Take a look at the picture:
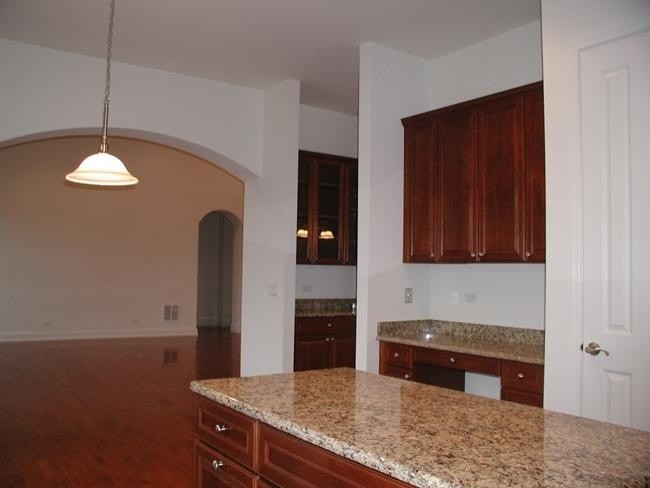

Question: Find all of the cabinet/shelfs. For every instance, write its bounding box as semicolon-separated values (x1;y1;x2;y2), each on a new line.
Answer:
498;353;545;413
296;147;358;268
294;308;357;373
191;395;422;488
521;75;547;267
434;83;526;267
396;107;439;267
376;337;465;393
410;343;502;379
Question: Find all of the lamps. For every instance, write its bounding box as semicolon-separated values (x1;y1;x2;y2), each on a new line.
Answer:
55;1;143;189
295;220;336;242
